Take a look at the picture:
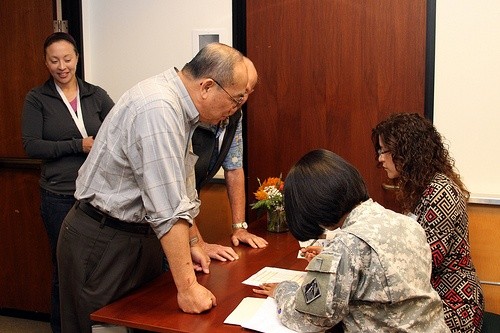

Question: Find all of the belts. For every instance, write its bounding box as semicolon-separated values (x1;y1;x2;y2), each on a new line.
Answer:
42;189;75;199
78;200;155;234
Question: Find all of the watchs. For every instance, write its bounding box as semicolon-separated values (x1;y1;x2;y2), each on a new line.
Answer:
232;222;248;231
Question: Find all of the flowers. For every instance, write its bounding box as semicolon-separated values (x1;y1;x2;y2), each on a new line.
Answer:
250;173;284;212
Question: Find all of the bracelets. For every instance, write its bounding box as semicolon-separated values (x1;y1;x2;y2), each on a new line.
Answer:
189;235;199;246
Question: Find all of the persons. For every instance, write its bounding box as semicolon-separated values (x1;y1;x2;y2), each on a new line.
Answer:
371;110;485;333
21;31;115;333
190;55;270;262
252;149;451;333
56;42;248;333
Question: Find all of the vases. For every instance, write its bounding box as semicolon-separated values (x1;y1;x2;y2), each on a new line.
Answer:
266;210;289;232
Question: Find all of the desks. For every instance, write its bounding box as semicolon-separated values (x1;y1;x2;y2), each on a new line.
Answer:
90;215;326;333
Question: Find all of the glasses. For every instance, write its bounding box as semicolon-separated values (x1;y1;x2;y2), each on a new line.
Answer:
377;149;392;156
200;77;242;109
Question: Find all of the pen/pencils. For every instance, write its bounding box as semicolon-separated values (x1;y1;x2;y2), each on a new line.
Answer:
301;239;317;256
